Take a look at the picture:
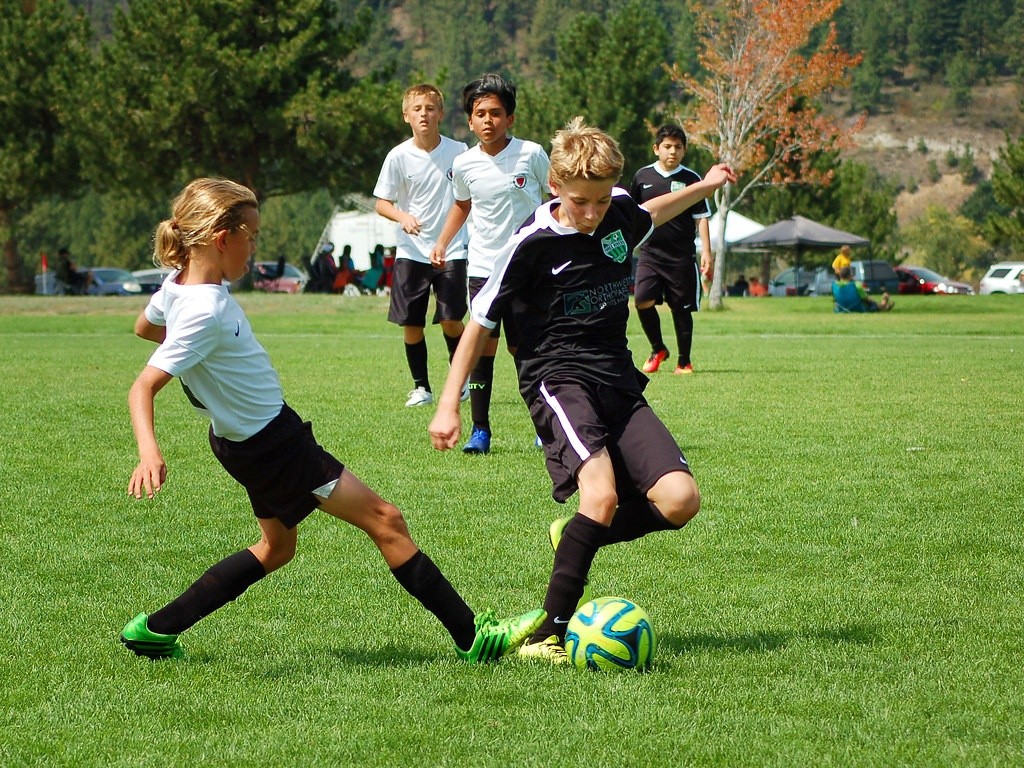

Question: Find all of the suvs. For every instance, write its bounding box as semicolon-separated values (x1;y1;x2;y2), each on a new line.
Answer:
979;260;1024;294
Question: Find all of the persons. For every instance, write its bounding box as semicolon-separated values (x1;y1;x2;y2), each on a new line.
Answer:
119;176;550;663
314;242;385;292
832;268;895;311
832;245;851;276
430;73;554;453
428;115;738;666
371;84;472;408
629;124;703;374
278;254;285;276
726;275;768;297
56;248;94;295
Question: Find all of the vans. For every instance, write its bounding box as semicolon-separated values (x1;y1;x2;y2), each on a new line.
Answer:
811;259;901;294
34;268;142;295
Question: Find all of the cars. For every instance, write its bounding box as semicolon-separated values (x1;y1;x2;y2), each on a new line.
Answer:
253;262;308;295
894;266;976;295
768;258;818;297
130;267;175;285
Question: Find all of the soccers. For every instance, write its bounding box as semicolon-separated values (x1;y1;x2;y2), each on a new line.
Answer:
564;596;657;672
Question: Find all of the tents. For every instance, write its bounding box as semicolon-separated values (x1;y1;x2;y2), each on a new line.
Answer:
694;208;766;283
728;214;874;296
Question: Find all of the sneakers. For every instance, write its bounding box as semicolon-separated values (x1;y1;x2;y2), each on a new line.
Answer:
675;363;693;375
120;613;185;659
641;346;669;373
405;386;432;408
517;633;569;664
461;373;471;402
455;609;548;664
462;427;489;454
550;515;591;613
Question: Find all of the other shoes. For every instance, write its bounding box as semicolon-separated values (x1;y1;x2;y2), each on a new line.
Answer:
885;300;895;311
881;293;889;305
